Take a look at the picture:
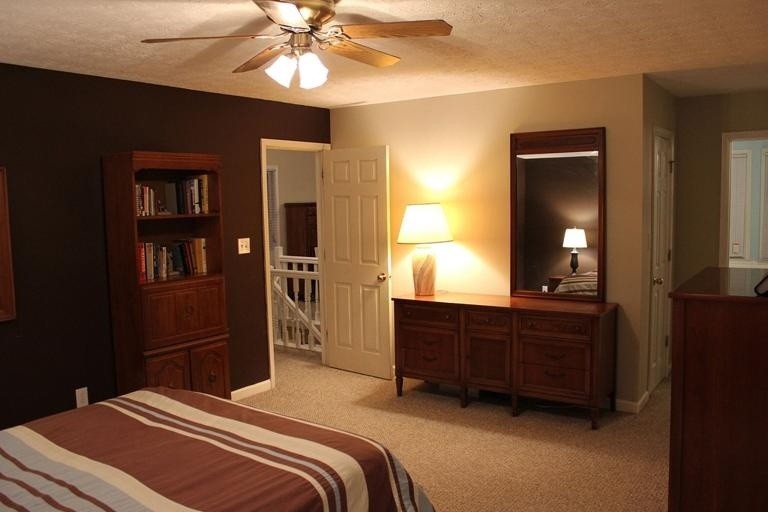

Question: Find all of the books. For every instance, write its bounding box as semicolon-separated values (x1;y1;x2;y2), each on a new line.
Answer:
138;237;208;285
135;174;210;217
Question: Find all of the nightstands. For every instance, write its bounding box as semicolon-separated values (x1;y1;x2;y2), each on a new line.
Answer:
547;275;564;292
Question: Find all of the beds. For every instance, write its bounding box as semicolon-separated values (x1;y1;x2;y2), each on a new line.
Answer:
554;269;597;297
0;386;437;512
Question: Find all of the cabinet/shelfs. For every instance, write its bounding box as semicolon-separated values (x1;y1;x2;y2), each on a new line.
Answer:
391;289;619;429
667;265;768;511
102;150;231;402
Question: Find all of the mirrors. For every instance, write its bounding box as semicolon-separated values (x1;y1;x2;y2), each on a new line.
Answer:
510;126;606;303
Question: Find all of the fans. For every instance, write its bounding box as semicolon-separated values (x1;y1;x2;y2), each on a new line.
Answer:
141;0;453;74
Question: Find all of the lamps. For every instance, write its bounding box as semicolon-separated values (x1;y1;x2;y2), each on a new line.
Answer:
396;201;454;296
563;226;588;273
264;47;330;90
